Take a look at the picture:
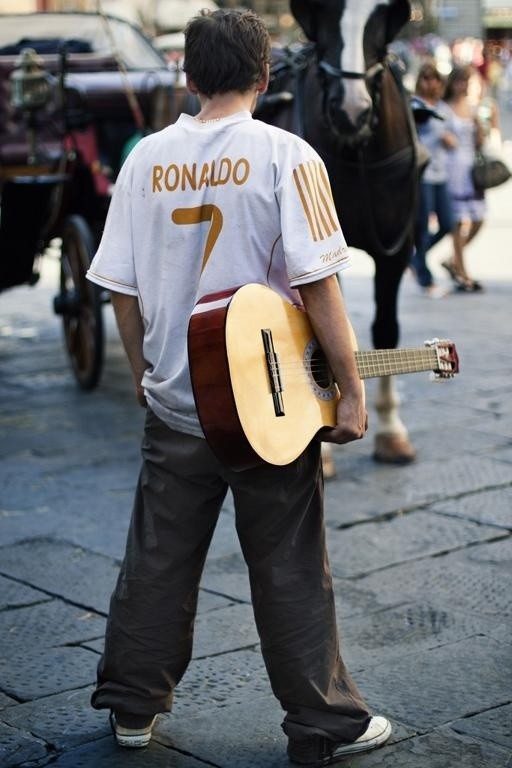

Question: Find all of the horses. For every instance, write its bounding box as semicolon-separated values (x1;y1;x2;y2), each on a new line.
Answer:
176;0;422;478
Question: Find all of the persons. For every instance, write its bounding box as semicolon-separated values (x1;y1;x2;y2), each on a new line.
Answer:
85;7;392;766
409;31;511;292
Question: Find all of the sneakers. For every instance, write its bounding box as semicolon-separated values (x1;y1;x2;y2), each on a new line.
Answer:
286;714;392;768
405;256;485;301
110;711;158;748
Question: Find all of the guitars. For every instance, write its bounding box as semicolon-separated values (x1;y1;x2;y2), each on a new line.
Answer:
188;284;459;471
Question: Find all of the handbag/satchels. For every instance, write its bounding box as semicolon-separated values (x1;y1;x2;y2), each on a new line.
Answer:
471;143;512;191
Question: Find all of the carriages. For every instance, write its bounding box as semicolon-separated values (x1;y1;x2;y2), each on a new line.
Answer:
0;0;442;482
